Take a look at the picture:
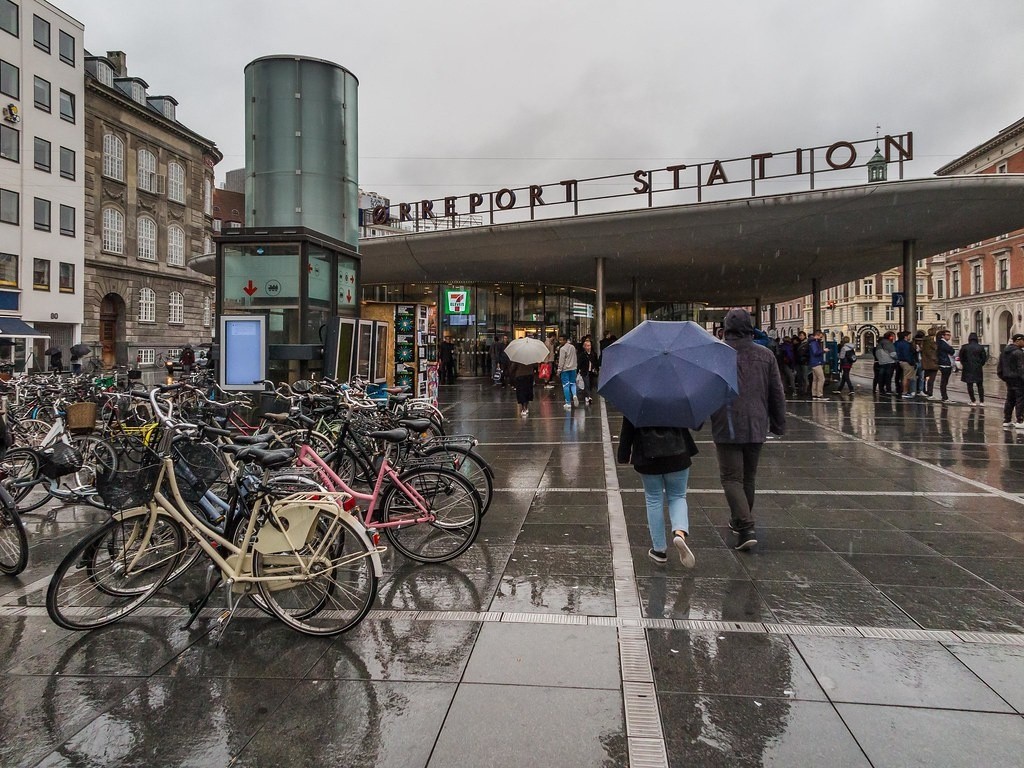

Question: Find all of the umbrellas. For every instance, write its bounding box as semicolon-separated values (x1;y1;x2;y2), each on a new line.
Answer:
69;344;91;356
45;346;62;355
597;320;739;429
504;337;550;365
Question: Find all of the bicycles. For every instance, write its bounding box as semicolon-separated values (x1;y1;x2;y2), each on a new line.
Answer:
0;352;496;639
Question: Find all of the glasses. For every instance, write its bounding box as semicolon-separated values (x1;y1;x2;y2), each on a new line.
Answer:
944;333;951;336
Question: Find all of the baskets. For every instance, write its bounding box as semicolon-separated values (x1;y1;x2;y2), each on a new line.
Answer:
257;395;291;419
35;442;84;480
94;434;164;510
164;440;227;502
125;405;151;427
65;402;96;433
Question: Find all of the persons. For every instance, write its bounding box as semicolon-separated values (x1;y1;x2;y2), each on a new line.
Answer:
70;354;83;377
481;330;617;391
179;343;195;373
200;347;214;374
510;362;533;416
137;355;141;368
440;336;455;385
556;333;579;408
959;333;987;406
617;415;699;569
691;310;786;550
873;328;956;404
997;334;1024;428
51;353;62;377
751;328;855;401
578;341;595;402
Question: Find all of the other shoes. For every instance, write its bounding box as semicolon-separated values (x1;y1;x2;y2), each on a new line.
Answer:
563;396;592;409
728;520;738;530
544;384;554;388
782;391;854;403
520;409;529;415
734;533;757;550
672;536;695;569
870;387;958;405
968;401;977;406
648;548;668;562
1003;419;1015;427
980;402;986;407
1015;421;1024;429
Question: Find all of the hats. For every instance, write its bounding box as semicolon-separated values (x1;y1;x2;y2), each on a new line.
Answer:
1013;334;1024;339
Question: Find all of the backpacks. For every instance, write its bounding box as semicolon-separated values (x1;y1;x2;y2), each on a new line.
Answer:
639;424;686;463
997;351;1018;382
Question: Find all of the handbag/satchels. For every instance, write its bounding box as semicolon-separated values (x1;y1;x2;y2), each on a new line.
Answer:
538;363;551;381
493;368;504;384
576;374;585;390
845;349;857;363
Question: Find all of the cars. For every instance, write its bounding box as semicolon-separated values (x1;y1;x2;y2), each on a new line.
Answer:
948;349;963;373
165;346;210;374
